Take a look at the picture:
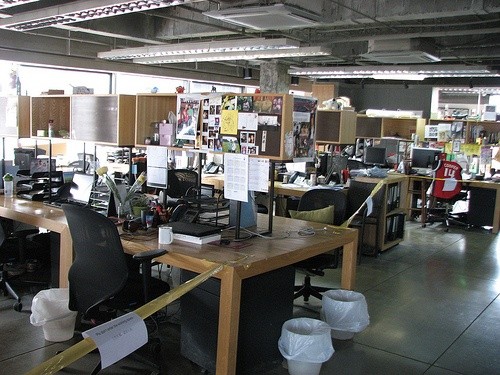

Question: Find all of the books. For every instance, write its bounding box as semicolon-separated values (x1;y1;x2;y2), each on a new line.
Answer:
157;222;221;245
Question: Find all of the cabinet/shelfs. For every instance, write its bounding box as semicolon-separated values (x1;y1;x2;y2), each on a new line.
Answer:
0;95;500;160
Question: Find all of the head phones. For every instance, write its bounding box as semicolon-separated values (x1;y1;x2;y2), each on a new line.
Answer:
122;220;149;232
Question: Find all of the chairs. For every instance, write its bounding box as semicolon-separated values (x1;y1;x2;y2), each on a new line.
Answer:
344;181;386;264
0;217;23;312
425;189;473;232
68;160;90;173
291;189;348;299
166;169;199;204
63;202;167;375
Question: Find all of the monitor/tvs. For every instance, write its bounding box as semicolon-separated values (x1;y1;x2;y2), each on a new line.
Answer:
364;147;387;166
410;148;443;170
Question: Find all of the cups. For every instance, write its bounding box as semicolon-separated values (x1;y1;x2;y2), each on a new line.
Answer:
158;227;173;245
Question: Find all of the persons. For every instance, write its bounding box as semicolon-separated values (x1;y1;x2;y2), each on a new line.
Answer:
273;98;281;112
203;99;256;154
295;112;315;147
178;103;193;127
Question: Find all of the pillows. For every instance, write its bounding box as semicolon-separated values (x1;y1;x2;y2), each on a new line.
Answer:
289;204;335;255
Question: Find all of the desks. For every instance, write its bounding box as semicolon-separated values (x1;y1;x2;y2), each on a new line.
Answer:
0;188;359;375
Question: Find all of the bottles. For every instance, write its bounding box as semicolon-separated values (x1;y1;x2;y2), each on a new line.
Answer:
3;173;14;198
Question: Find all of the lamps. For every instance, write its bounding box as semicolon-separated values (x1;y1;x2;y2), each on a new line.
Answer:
96;37;333;65
288;66;500;80
0;1;196;31
243;68;252;80
291;77;300;86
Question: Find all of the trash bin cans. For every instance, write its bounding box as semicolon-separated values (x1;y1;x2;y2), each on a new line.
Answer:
42;288;76;342
324;290;364;340
282;317;331;375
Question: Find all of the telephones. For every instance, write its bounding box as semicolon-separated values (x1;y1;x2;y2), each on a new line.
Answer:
289;171;306;183
169;203;200;224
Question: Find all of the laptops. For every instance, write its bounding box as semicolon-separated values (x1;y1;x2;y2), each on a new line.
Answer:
157;220;221;237
44;173;97;210
